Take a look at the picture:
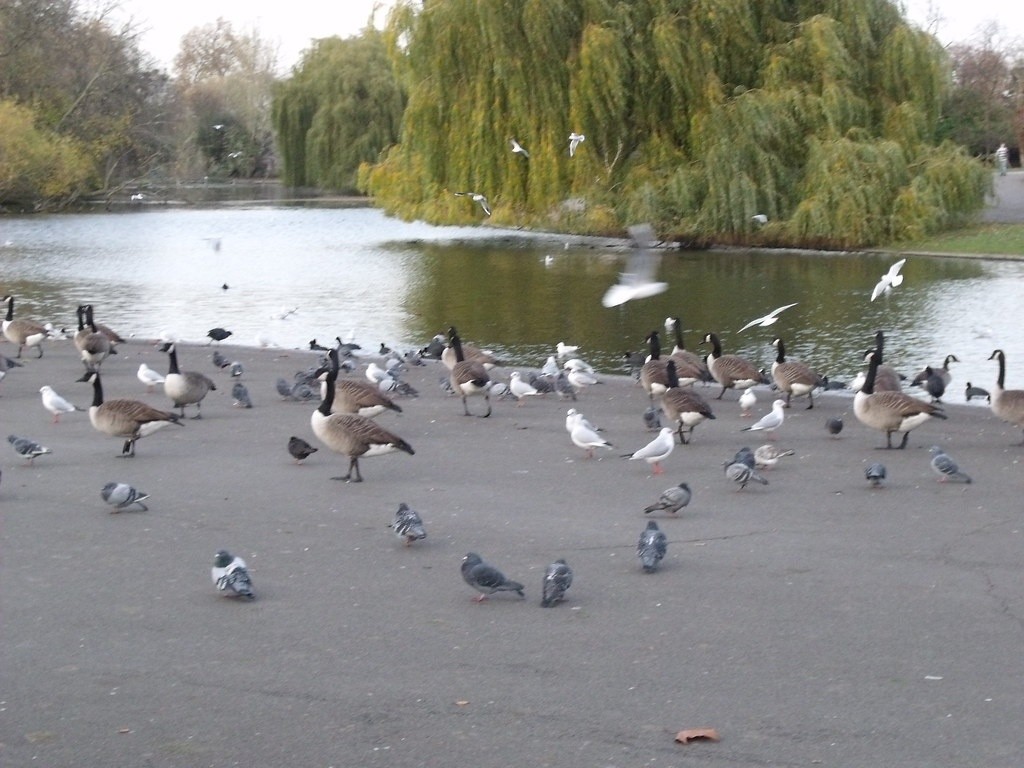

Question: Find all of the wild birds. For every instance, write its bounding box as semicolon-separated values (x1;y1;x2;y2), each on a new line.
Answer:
637;479;692;574
510;140;530;159
863;448;974;490
543;242;572;268
870;257;907;302
568;132;585;158
129;123;244;205
442;326;497;418
849;330;1024;451
0;292;218;423
5;435;52;465
642;311;848;445
211;550;256;600
0;240;13;249
310;368;416;483
394;503;428;546
487;343;795;493
288;437;318;465
43;320;448;411
455;190;491;217
74;370;186;459
101;481;151;514
37;385;87;424
459;550;574;609
752;214;768;223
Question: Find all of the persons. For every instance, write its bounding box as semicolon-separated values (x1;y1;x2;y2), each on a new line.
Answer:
996;142;1008;177
